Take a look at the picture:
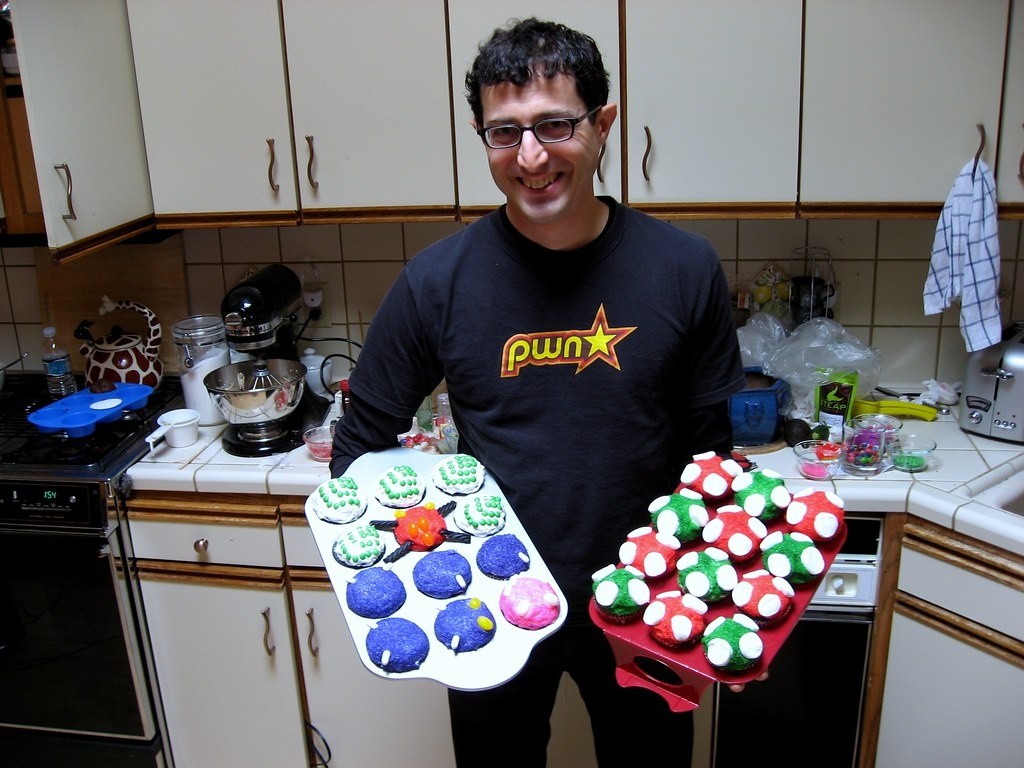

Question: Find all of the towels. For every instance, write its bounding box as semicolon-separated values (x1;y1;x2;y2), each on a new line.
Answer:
922;155;1005;354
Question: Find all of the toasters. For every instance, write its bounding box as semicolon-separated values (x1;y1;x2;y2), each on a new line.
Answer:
959;321;1024;443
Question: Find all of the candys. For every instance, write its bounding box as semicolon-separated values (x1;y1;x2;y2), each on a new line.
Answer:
849;443;880;467
399;433;432;451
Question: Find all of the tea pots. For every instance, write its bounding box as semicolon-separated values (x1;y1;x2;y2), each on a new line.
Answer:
73;294;164;397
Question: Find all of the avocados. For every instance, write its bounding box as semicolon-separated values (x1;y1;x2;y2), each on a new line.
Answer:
783;419;813;446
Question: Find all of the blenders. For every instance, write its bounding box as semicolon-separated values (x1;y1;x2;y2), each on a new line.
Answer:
201;263;332;457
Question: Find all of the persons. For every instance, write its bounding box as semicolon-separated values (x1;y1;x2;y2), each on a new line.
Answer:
329;19;748;768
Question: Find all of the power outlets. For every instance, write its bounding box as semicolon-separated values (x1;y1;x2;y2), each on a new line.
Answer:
304;282;332;328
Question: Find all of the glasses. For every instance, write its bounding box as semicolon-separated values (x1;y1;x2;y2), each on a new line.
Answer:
474;105;601;150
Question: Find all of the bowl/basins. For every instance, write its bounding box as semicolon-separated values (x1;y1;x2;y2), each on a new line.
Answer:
854;412;902;458
303;424;336;461
794;439;846;481
300;348;332;399
885;433;937;473
441;423;459;453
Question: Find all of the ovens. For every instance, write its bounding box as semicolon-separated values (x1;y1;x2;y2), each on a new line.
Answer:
0;518;170;768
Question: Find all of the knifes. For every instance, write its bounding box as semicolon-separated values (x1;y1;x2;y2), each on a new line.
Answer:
876;386;950;415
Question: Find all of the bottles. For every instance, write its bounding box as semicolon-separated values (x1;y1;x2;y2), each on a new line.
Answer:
39;327;77;403
340;379;355;414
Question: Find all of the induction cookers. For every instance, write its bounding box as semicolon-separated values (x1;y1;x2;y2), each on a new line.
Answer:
0;368;187;531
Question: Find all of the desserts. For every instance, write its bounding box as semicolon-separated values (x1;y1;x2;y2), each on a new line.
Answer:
308;453;560;673
590;451;845;673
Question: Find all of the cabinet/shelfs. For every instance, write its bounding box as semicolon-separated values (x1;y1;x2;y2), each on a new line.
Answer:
120;496;453;768
858;518;1024;768
0;0;1024;266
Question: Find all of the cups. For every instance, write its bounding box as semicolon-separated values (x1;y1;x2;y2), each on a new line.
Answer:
169;313;230;428
841;418;886;477
438;394;454;429
